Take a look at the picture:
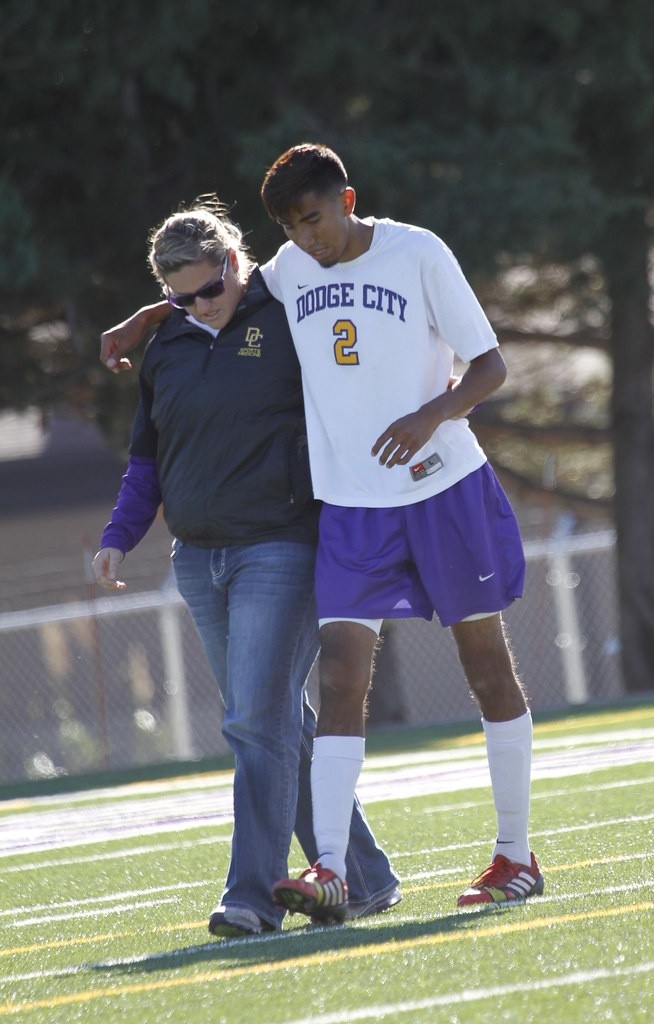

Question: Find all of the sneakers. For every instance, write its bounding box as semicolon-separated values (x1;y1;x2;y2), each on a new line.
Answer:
313;878;399;930
271;853;349;925
456;853;546;908
209;902;261;937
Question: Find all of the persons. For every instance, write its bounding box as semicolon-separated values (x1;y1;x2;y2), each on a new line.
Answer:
99;143;544;925
92;190;474;940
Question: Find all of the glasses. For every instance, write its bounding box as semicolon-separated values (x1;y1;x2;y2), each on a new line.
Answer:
167;255;230;309
